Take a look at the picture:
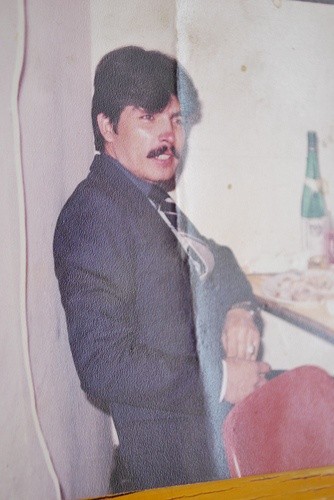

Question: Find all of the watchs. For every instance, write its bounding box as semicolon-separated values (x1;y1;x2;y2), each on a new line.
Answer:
232;301;263;328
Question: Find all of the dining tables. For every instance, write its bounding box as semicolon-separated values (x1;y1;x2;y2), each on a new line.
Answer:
246;269;334;346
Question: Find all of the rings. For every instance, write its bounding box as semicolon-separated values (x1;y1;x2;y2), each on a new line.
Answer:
247;345;254;353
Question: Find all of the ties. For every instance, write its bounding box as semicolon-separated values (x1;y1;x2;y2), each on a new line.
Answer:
148;183;215;282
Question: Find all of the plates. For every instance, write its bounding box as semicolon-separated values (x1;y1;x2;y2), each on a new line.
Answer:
261;273;334;318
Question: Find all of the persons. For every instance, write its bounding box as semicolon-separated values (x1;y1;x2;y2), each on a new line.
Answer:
53;46;272;492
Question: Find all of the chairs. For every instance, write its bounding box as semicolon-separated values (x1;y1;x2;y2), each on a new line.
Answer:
222;365;334;479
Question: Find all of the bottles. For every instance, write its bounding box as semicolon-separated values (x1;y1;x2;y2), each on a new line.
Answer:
301;132;330;271
